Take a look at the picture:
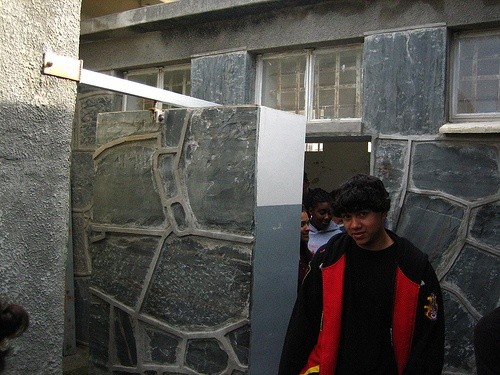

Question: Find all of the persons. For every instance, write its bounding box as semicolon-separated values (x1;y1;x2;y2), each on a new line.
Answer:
278;174;445;375
297;186;346;296
